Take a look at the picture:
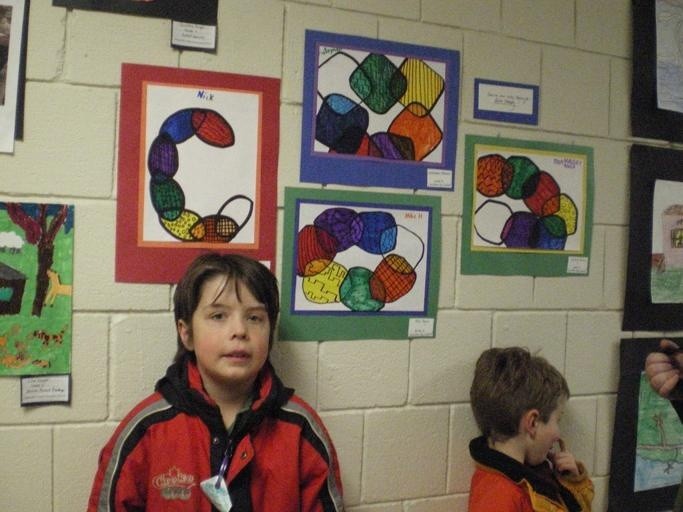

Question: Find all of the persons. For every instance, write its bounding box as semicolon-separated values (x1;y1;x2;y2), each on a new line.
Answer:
466;345;596;512
84;252;347;512
646;339;682;429
